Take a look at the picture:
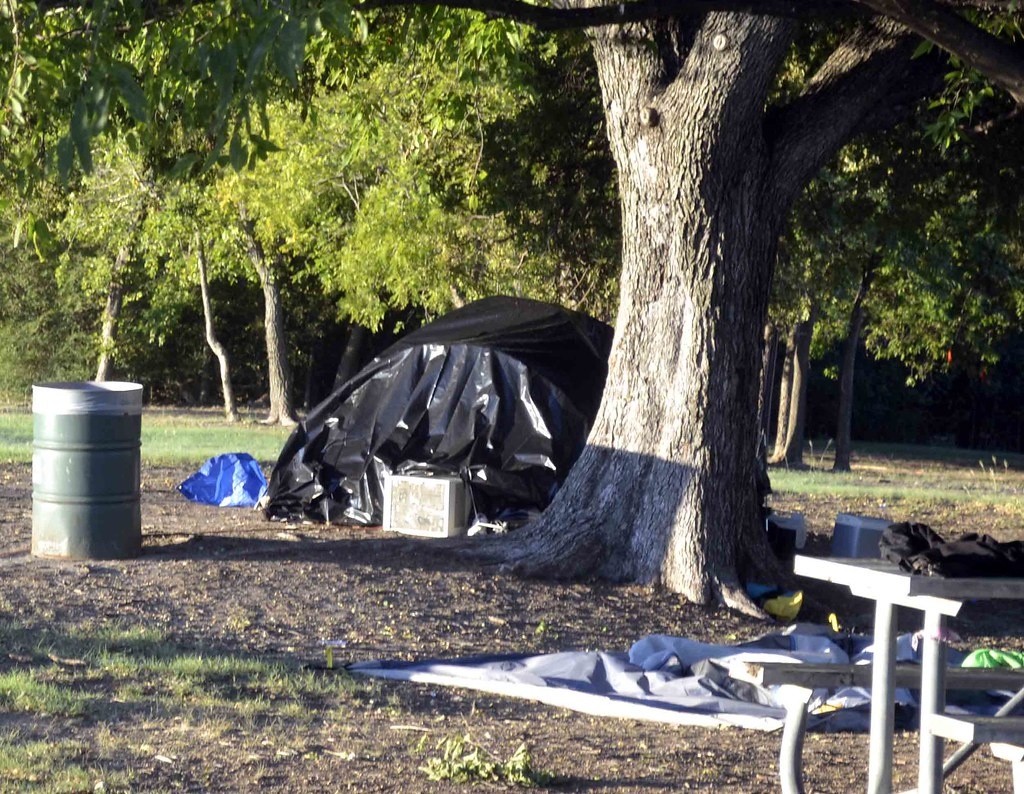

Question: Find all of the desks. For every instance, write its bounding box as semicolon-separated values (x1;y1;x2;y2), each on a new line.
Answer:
793;554;1024;794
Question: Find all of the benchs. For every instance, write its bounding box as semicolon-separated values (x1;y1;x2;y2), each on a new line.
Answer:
728;659;1024;794
928;714;1024;794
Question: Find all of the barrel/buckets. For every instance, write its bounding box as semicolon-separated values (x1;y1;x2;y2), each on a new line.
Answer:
830;513;895;560
32;380;143;561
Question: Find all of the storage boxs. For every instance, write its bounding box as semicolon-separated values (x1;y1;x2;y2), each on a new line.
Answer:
830;513;893;559
384;475;470;537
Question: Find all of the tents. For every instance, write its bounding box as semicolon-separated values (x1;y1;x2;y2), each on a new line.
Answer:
257;294;613;532
